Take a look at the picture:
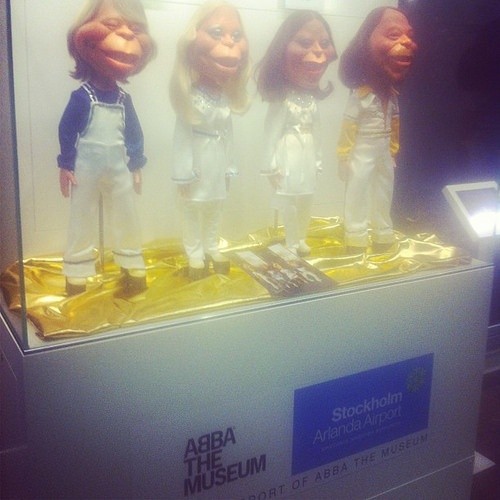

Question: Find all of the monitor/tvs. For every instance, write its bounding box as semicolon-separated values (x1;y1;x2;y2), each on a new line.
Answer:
443;181;499;244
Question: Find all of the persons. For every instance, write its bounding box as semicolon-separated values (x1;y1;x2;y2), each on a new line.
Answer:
254;7;339;258
57;0;158;297
332;4;417;255
159;0;260;278
